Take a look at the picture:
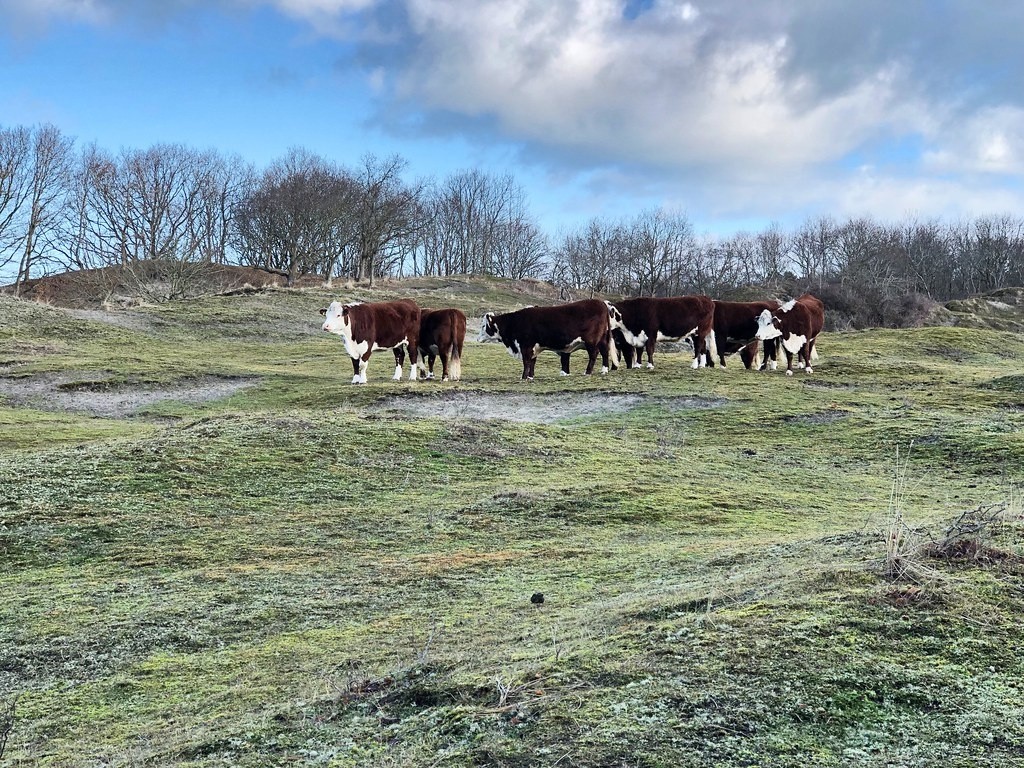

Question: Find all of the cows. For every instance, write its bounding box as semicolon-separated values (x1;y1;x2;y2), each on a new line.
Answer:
319;299;467;383
477;295;824;376
